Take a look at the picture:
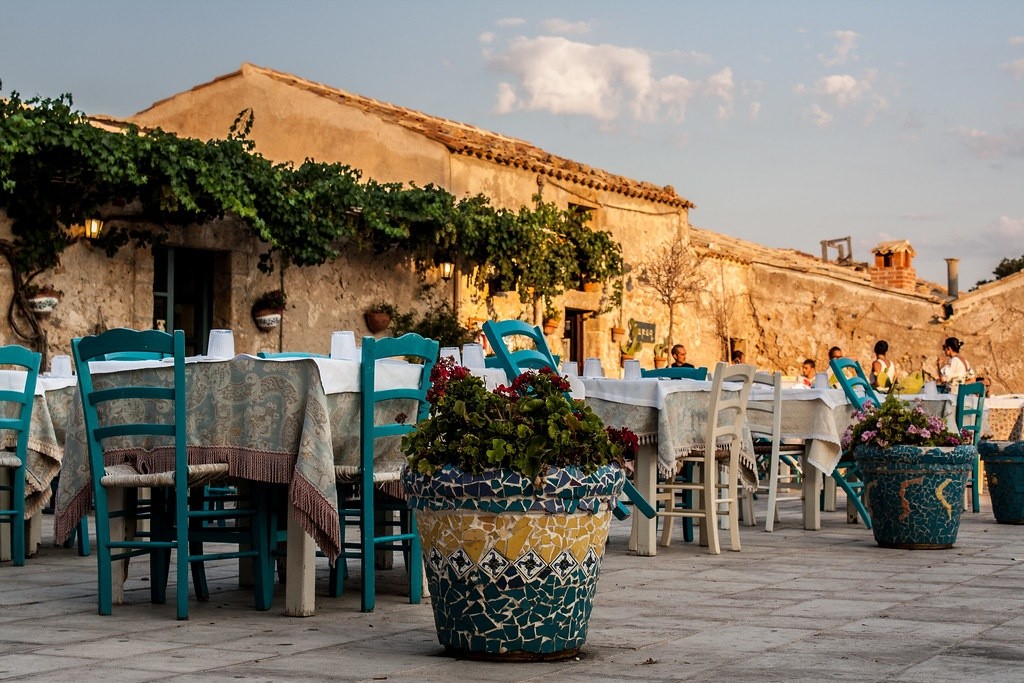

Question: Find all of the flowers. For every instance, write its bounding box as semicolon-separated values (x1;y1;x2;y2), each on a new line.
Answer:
252;289;287;312
25;285;63;300
841;396;972;449
395;358;639;477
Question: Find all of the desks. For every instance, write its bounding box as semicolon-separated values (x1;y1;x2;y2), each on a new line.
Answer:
0;370;77;560
53;355;587;618
751;386;866;532
873;397;977;511
576;377;742;555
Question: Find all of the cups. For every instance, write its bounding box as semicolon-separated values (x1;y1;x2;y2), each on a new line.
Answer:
462;343;485;369
50;354;72;378
815;373;829;389
924;382;937;395
207;329;234;360
440;346;461;367
330;331;357;361
583;358;601;377
562;361;578;377
624;360;641;380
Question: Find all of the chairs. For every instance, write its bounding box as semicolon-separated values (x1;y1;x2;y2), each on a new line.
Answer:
0;314;987;624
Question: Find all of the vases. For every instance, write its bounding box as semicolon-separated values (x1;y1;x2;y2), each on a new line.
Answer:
253;310;281;332
28;297;58;313
976;440;1024;525
855;444;973;550
402;461;623;662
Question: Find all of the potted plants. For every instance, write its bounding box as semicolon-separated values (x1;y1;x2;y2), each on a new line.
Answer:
619;319;643;368
543;307;560;333
365;302;393;333
652;338;672;370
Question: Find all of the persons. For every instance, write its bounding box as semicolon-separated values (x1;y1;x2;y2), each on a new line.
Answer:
730;351;744;365
939;336;974;392
871;339;896;390
825;345;846;377
673;345;695;379
801;359;815;388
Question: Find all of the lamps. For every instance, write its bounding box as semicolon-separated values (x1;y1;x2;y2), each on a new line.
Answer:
434;243;456;282
83;210;104;239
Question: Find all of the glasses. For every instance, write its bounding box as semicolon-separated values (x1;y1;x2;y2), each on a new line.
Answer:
943;344;948;350
